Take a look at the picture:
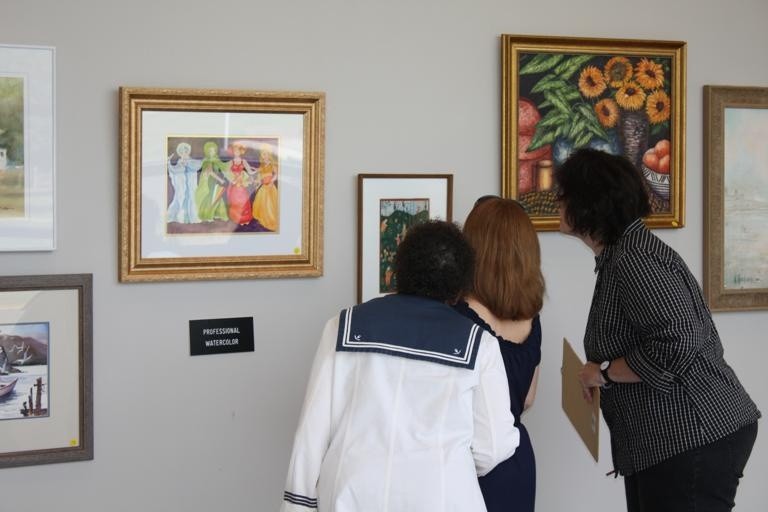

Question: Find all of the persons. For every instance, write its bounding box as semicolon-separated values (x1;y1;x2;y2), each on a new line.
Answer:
280;219;521;512
554;147;762;511
452;195;536;512
167;139;279;233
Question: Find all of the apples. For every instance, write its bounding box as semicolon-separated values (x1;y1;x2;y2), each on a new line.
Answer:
643;140;671;174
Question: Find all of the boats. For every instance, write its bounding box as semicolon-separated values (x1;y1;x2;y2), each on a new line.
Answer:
0;375;21;396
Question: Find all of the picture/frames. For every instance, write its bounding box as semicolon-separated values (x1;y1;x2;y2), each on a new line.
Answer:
703;82;768;314
354;172;454;305
0;276;94;467
115;87;326;282
0;42;59;255
497;31;688;235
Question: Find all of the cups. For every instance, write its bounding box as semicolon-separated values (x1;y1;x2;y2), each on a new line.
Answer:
535;158;555;193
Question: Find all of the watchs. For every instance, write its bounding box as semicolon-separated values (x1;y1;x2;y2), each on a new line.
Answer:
599;359;612;384
